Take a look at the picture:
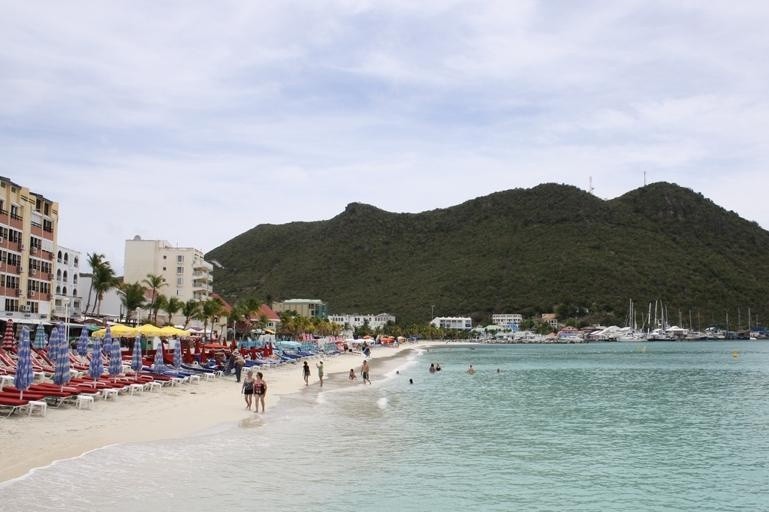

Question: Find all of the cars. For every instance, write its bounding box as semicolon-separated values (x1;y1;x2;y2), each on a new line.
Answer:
558;336;585;343
521;334;542;344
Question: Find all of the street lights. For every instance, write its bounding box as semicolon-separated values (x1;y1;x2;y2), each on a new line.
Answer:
431;305;435;318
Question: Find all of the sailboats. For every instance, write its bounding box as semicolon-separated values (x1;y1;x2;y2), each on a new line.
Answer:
607;298;769;342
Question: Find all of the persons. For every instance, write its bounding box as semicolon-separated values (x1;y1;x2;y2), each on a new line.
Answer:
466;364;475;374
349;359;371;385
316;360;324;386
362;341;371;357
429;364;440;372
409;379;413;384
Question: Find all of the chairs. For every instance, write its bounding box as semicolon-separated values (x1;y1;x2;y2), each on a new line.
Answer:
0;343;341;419
